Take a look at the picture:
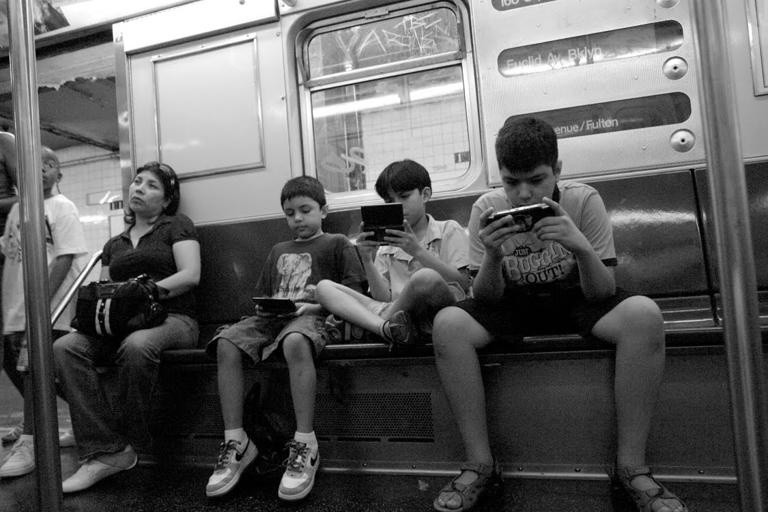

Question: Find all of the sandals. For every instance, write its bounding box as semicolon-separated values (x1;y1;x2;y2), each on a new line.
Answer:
380;310;418;343
433;458;501;512
325;315;370;342
611;464;688;512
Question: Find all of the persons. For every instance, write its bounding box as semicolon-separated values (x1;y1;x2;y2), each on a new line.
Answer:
204;175;367;502
314;158;472;352
431;116;689;512
50;164;200;494
0;131;25;442
0;146;88;477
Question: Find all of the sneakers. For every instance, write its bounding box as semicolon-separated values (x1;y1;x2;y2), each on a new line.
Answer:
0;434;38;476
62;445;137;494
278;439;321;500
207;436;258;496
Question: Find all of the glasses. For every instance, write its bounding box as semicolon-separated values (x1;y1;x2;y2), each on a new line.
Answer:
146;160;175;187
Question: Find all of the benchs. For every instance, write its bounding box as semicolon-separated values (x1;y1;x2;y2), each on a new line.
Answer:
58;171;741;490
693;156;768;345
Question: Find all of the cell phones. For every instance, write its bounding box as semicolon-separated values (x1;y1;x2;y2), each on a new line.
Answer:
252;295;296;316
493;203;551;234
360;202;405;243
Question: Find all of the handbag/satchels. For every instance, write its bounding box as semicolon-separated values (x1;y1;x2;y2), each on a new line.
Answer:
76;275;168;338
